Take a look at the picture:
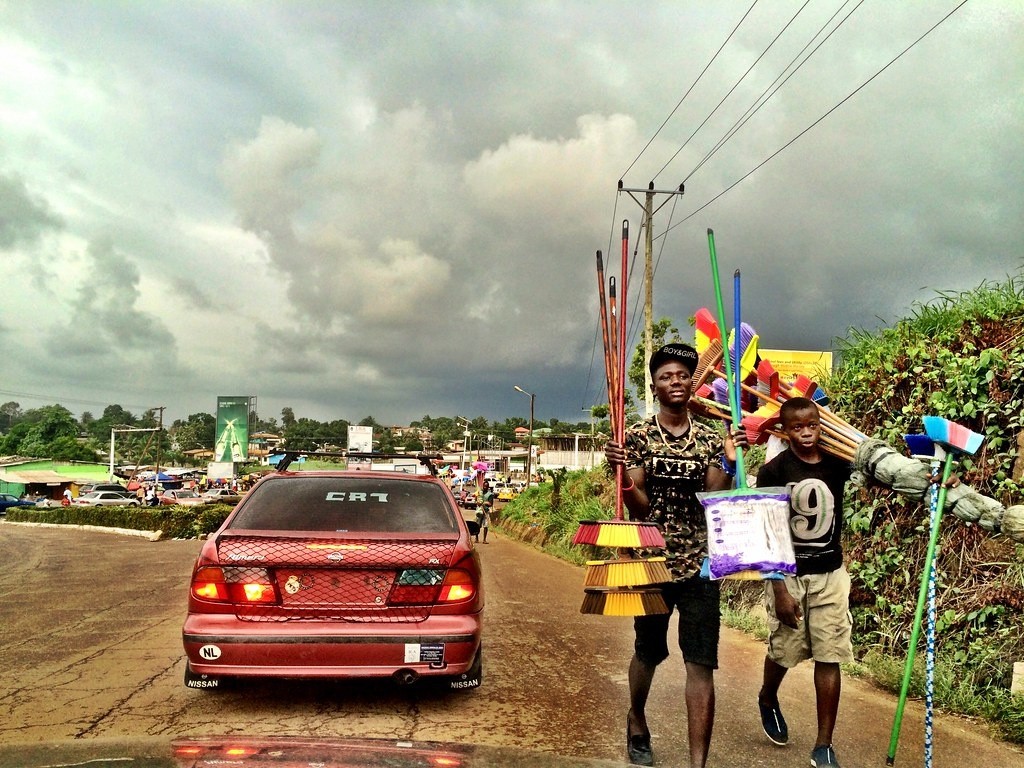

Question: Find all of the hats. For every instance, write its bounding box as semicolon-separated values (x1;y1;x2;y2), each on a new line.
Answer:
650;343;699;379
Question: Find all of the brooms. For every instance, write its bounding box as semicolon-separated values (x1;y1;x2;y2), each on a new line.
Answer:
686;307;874;463
885;415;986;768
698;227;788;579
568;218;674;619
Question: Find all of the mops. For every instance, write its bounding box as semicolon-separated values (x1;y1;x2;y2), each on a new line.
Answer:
701;269;797;577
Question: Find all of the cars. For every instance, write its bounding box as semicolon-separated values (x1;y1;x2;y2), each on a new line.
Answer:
465;494;480;509
512;483;523;493
451;488;471;499
0;493;35;512
203;489;242;504
182;447;484;693
79;484;130;497
160;490;205;507
485;477;502;487
528;481;538;487
81;431;87;437
71;491;140;509
498;488;521;502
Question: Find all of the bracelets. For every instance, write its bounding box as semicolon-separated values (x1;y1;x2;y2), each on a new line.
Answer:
620;477;635;491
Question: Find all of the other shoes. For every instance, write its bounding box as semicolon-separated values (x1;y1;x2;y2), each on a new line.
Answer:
475;538;478;543
626;708;653;766
759;689;788;745
811;743;841;768
482;541;489;544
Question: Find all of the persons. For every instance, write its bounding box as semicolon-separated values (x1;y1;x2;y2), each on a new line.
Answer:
63;487;72;501
755;398;962;767
605;344;751;768
475;481;493;544
181;473;255;497
137;486;145;504
60;495;70;506
19;492;26;498
149;494;159;506
146;487;154;505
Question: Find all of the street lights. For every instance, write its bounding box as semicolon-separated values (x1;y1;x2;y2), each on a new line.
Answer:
514;385;535;489
456;423;468;492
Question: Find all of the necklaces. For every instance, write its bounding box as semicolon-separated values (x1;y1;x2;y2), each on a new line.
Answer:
655;415;693;453
